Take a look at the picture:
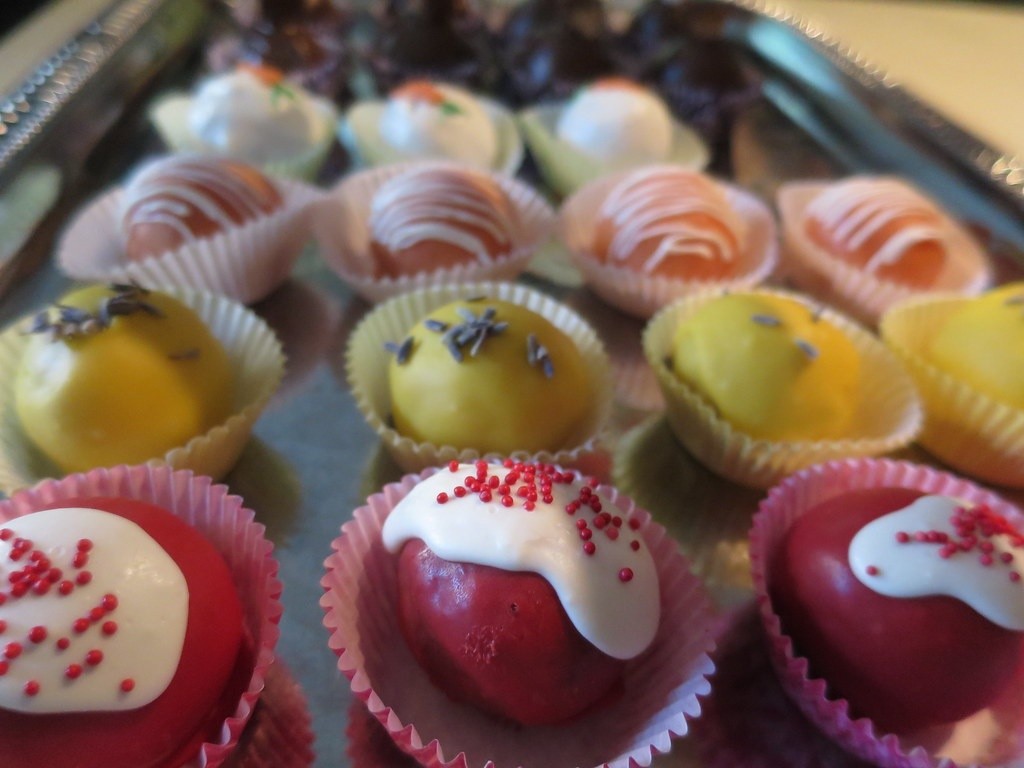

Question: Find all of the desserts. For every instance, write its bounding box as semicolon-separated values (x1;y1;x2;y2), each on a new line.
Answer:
0;0;1024;768
